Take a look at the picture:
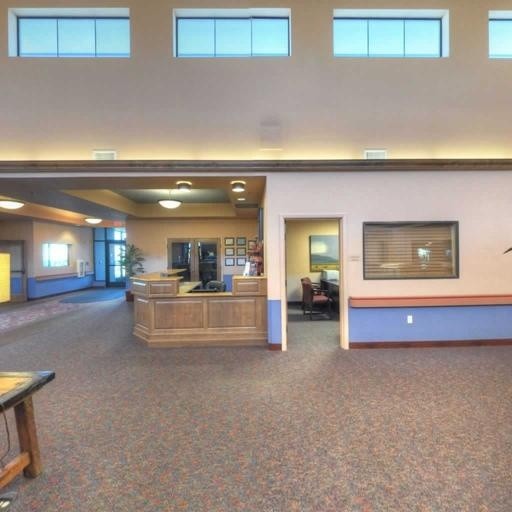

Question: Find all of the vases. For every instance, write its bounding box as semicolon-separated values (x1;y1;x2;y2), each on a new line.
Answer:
256;259;263;276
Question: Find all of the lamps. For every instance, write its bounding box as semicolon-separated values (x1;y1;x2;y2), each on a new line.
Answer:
230;181;247;193
176;181;193;193
83;216;103;225
0;200;25;211
158;199;183;210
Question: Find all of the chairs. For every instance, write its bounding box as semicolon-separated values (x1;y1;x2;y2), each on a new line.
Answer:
300;277;326;314
302;282;334;321
205;279;227;291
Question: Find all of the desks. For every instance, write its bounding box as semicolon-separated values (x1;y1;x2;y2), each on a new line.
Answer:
320;278;339;298
0;371;57;491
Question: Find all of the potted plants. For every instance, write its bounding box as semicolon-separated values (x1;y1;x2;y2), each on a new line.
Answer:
116;241;146;301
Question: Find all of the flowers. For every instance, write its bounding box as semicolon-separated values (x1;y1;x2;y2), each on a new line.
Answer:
246;239;263;264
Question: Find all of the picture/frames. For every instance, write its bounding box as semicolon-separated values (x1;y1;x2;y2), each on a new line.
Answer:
224;235;257;266
